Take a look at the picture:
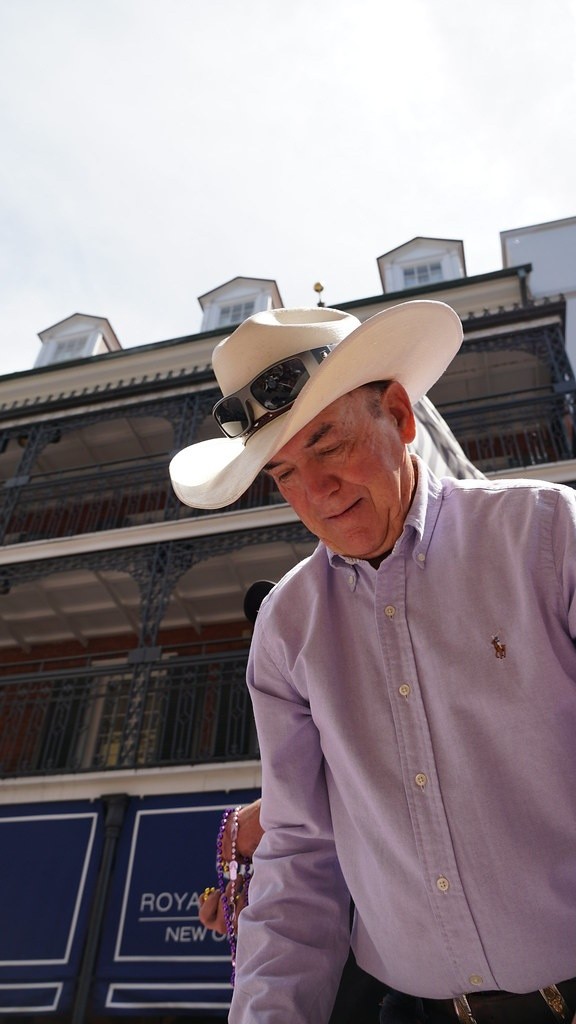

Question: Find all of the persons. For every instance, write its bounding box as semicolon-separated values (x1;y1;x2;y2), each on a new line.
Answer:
171;298;576;1024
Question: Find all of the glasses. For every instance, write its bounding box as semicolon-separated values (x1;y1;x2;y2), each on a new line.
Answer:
212;347;332;440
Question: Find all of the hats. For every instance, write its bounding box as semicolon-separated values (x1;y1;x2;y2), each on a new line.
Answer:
168;300;464;509
244;580;278;625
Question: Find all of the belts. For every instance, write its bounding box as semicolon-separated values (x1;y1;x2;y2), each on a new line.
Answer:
423;980;576;1024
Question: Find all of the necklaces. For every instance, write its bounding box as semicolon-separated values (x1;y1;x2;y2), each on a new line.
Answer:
215;805;241;986
